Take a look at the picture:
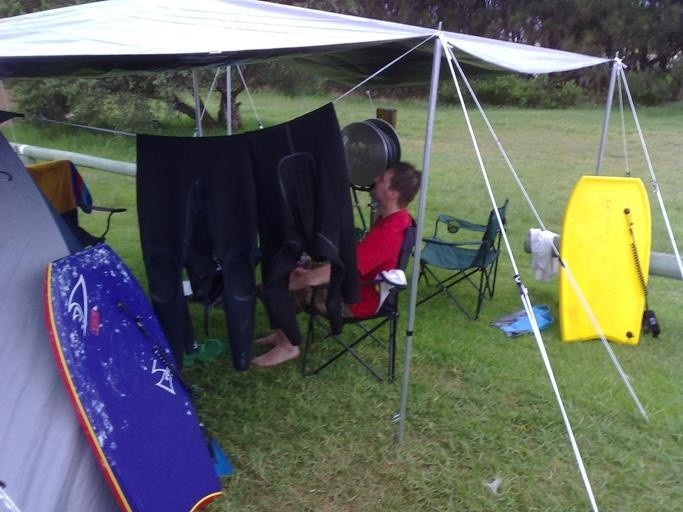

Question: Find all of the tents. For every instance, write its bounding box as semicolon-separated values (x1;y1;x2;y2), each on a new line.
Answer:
0;110;233;512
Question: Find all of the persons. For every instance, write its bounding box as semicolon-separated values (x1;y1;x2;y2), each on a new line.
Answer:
251;161;421;367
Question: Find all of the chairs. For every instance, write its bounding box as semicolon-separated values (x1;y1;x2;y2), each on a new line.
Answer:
298;196;509;379
24;158;128;248
